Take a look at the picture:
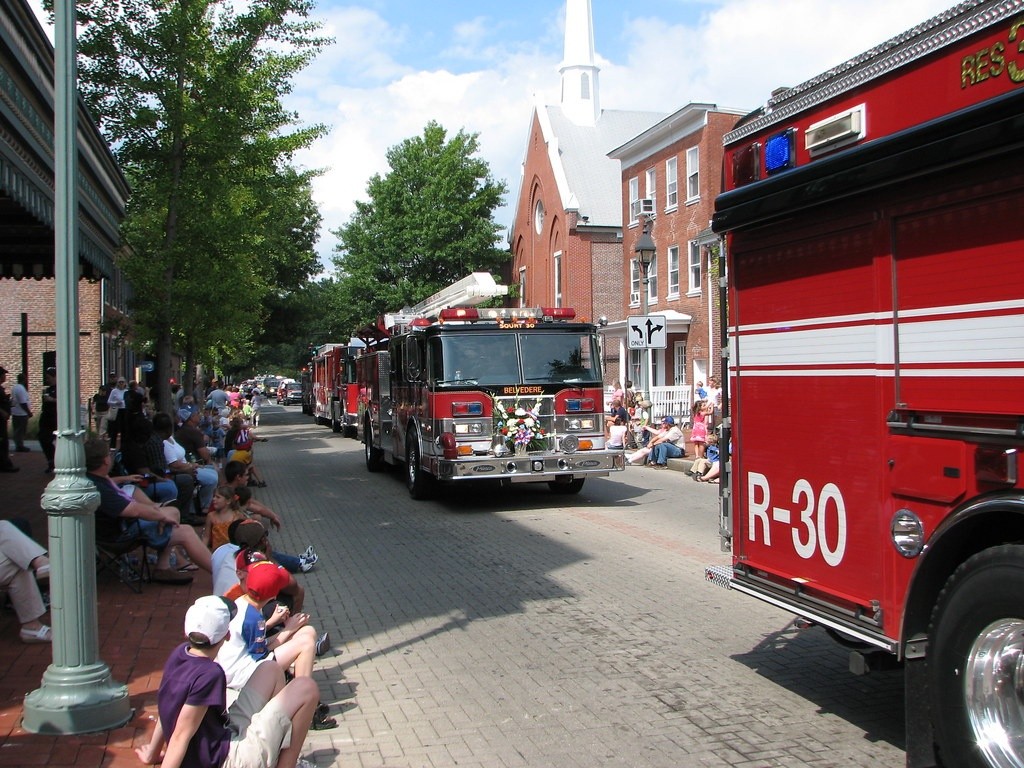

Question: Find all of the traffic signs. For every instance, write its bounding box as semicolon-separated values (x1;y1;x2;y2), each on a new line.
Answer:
627;314;668;350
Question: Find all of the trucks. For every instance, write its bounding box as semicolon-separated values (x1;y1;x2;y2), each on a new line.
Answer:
247;373;302;406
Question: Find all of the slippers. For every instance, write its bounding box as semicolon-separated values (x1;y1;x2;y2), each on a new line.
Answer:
177;563;199;571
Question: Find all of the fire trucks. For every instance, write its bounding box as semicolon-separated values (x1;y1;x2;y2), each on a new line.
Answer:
711;0;1024;768
301;272;625;503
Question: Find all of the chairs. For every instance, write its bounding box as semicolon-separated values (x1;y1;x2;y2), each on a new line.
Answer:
95;445;217;596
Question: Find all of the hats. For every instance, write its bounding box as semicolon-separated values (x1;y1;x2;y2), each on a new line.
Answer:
236;549;269;571
662;416;674;423
177;404;196;422
236;519;268;548
247;561;289;602
184;595;238;644
0;367;8;374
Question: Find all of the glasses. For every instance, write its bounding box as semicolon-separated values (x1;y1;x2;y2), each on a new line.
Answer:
119;381;125;383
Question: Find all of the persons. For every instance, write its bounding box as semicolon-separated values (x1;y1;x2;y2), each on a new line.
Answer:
0;366;336;768
603;377;685;470
686;375;732;484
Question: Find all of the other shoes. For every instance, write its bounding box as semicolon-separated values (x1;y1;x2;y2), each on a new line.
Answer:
45;462;54;473
181;513;206;526
0;466;20;473
152;567;193;585
309;703;336;730
16;446;29;452
258;481;267;488
316;633;330;655
200;509;208;516
247;480;257;487
646;461;717;484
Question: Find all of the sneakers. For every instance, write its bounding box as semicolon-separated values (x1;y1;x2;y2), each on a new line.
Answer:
298;546;314;561
300;554;319;572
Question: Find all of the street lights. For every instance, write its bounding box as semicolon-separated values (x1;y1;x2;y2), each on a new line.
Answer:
633;222;656;427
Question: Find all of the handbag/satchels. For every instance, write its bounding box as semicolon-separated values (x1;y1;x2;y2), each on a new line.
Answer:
106;404;119;421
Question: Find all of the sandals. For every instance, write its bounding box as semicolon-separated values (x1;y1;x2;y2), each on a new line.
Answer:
36;564;50;585
19;623;53;643
